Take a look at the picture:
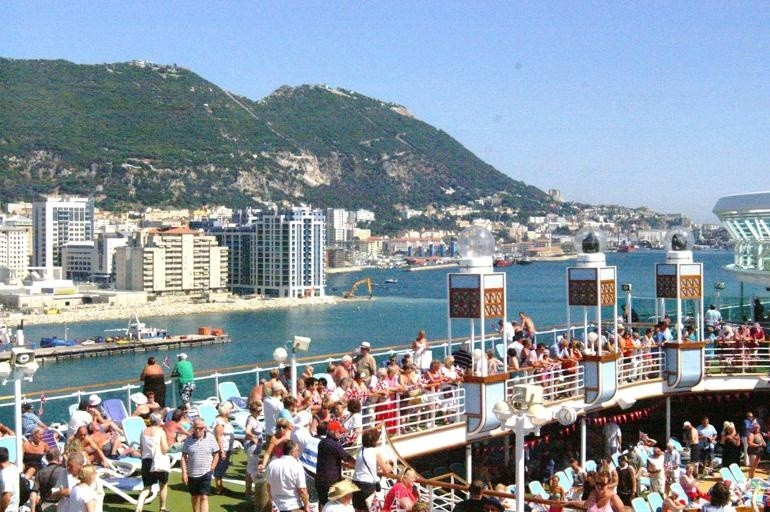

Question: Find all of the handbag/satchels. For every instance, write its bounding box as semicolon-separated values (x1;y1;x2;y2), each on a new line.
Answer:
150;450;172;473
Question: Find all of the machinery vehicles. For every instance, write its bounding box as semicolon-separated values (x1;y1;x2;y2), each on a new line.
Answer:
342;277;374;302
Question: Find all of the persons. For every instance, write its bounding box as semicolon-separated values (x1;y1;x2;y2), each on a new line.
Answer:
132;299;769;511
0;395;141;510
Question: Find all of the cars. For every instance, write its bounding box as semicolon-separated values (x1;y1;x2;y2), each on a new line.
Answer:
81;339;96;347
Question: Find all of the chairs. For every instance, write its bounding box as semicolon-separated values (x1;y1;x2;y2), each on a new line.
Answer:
312;372;336;391
0;382;265;505
507;439;746;512
496;344;506;358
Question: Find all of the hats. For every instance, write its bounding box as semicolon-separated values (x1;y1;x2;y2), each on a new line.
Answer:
129;392;148;405
176;352;188;361
87;393;102;405
326;418;346;433
360;341;370;348
326;477;360;502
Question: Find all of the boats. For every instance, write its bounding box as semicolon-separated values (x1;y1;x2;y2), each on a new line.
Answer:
0;317;37;351
617;244;639;253
39;335;104;347
104;312;170;338
492;252;516;267
385;279;398;283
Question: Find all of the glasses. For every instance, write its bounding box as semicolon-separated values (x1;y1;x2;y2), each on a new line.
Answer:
276;424;289;429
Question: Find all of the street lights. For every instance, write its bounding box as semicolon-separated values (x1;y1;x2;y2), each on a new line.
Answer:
0;347;40;477
491;385;549;511
273;335;312;399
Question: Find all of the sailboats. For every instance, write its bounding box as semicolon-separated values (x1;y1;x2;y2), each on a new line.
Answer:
517;252;532;264
